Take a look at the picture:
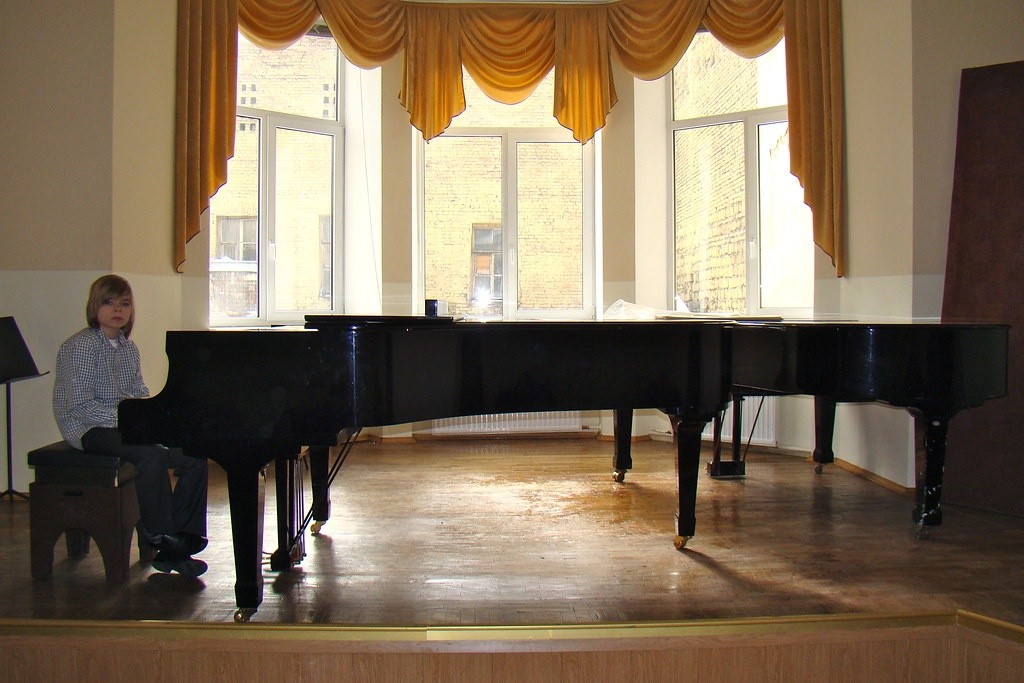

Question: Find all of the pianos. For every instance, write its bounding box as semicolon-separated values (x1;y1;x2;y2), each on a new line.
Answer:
610;309;1012;552
116;306;746;625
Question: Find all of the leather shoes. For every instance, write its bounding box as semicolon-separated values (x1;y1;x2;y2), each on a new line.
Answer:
152;550;209;578
142;530;209;556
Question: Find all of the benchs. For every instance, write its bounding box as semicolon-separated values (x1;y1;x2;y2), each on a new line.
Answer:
25;441;137;582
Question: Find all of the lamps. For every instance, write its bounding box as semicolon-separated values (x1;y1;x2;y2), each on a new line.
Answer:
0;315;42;505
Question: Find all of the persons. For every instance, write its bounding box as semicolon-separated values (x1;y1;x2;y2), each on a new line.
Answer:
51;274;209;578
